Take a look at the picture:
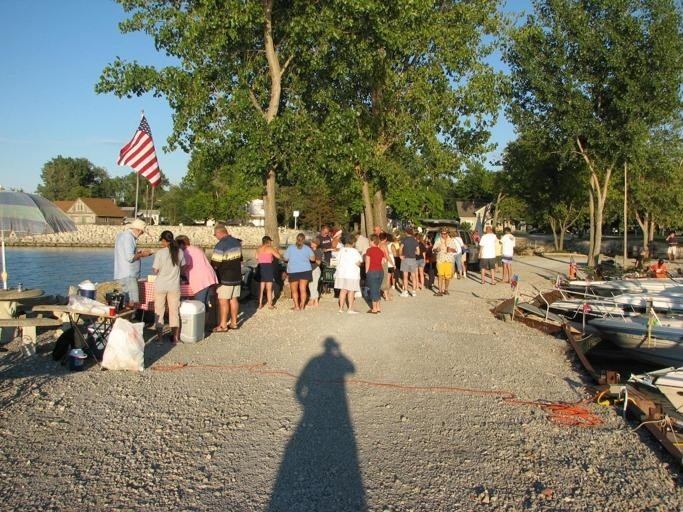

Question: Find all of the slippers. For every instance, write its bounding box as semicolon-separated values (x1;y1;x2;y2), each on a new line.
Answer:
154;338;164;345
481;282;496;284
366;310;381;314
170;339;185;344
291;307;305;311
268;306;278;310
258;303;264;308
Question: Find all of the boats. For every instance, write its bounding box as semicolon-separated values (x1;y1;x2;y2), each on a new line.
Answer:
535;271;683;474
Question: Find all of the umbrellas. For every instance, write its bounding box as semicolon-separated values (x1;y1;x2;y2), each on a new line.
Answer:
0;191;79;291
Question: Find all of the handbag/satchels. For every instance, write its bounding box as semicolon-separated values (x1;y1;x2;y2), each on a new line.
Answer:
461;245;467;255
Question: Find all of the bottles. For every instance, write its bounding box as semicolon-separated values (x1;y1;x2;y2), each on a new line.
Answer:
106;288;134;310
17;283;23;293
555;272;559;288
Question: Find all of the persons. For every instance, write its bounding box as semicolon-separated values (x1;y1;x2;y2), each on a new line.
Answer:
648;259;666;273
254;236;282;309
175;235;220;324
664;232;677;261
236;239;242;247
306;224;515;314
210;225;243;333
113;218;154;308
150;229;186;344
282;233;316;310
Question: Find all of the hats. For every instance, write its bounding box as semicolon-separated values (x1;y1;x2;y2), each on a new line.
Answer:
125;218;147;233
332;227;342;239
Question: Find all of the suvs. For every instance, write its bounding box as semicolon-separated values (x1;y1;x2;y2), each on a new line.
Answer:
416;215;480;272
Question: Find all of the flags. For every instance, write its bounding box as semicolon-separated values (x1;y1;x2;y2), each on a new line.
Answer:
114;115;161;187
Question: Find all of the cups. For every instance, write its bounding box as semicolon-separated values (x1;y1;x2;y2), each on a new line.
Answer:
447;245;450;252
108;301;120;316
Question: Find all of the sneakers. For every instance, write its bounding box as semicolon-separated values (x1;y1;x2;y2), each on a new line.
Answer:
399;290;410;292
411;290;417;297
339;308;359;314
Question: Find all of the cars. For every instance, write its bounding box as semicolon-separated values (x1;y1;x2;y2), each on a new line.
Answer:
222;219;254;226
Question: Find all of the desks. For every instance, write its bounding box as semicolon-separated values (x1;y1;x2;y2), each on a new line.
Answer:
128;277;195;326
60;300;137;370
0;287;44;314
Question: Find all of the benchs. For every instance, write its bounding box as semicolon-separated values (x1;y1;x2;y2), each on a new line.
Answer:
28;303;71;317
0;317;62;354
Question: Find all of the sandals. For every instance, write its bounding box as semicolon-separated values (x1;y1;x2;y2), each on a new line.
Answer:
207;323;239;332
432;289;450;296
